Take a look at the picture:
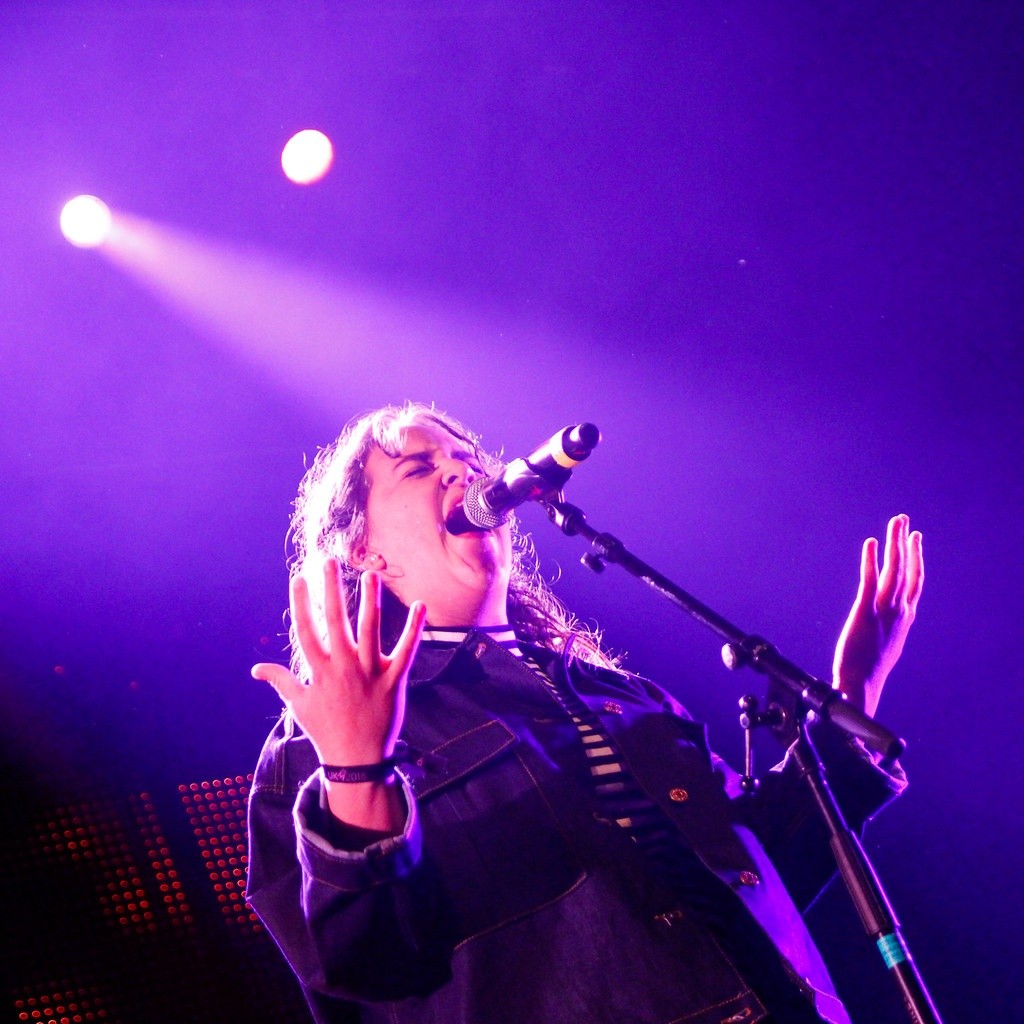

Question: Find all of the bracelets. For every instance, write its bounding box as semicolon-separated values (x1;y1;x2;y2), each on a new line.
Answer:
319;755;402;783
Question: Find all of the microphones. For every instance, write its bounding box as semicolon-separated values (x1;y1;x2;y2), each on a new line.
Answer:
462;422;600;527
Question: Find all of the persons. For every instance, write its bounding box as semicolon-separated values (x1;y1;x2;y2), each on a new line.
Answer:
243;404;922;1024
